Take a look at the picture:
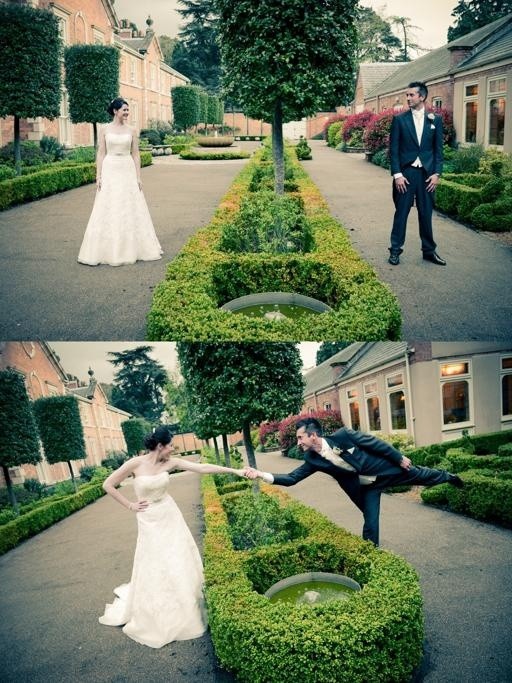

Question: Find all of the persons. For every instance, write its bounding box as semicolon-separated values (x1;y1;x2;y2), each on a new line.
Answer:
99;425;250;648
243;417;465;548
76;95;165;266
388;81;447;265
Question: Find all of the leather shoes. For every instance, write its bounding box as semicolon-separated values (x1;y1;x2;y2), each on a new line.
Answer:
389;254;399;265
423;251;446;266
448;473;464;489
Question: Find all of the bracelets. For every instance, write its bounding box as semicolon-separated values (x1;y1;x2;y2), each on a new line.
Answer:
128;503;132;510
